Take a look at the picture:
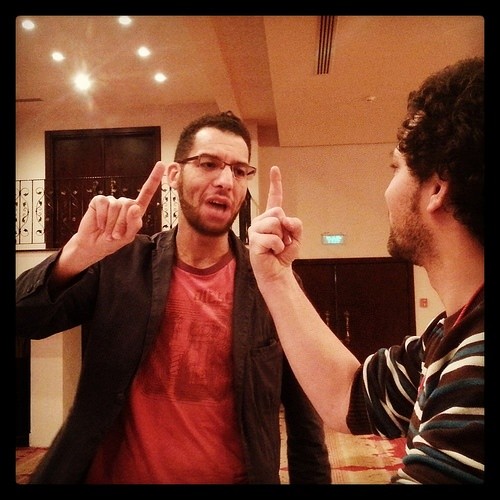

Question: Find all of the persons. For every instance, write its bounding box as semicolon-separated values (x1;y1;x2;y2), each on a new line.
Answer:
15;110;331;487
247;54;487;484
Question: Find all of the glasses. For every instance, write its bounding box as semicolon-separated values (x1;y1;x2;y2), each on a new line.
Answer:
181;152;257;181
